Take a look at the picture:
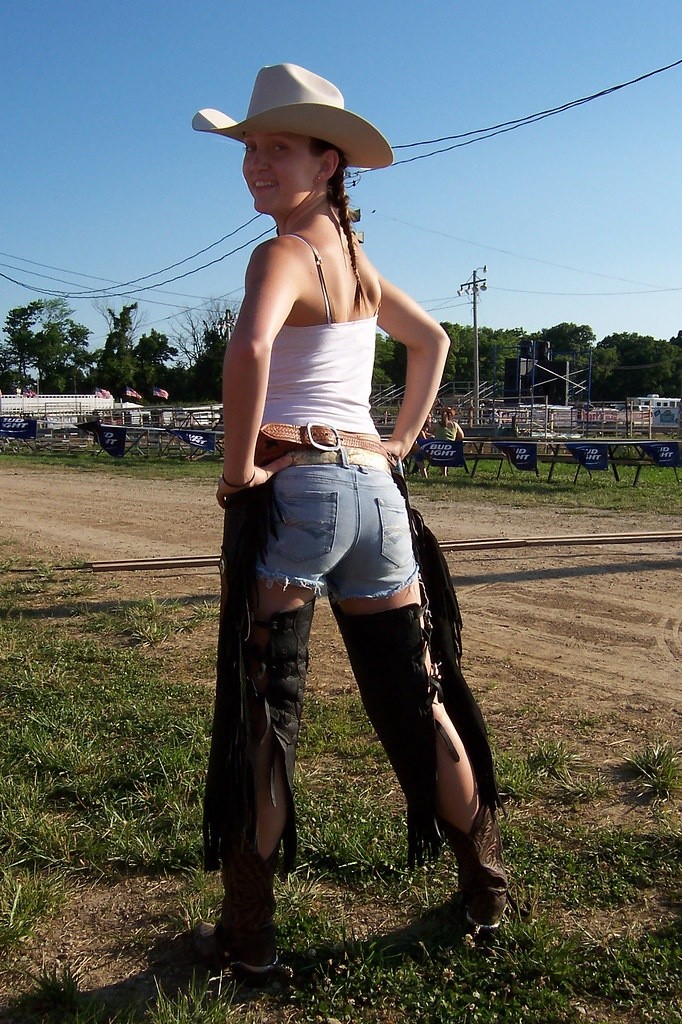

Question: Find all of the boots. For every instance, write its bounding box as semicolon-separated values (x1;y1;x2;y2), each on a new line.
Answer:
436;800;509;930
190;831;282;971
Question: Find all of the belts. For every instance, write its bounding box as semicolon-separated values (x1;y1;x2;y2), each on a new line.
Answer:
261;422;397;467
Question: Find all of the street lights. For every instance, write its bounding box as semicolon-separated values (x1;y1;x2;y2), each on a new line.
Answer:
456;266;488;424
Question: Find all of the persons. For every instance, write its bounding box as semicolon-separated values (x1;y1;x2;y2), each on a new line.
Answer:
190;64;511;973
26;389;35;398
411;409;465;475
380;412;392;423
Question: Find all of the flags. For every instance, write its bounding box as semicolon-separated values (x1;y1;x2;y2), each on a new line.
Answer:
95;386;109;399
125;385;142;398
152;386;169;400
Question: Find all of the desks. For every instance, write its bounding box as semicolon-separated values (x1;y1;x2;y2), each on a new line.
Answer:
0;421;682;457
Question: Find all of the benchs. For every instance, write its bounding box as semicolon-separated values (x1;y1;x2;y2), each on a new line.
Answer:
0;437;682;487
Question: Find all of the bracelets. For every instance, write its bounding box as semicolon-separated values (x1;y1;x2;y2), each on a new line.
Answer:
222;471;255;488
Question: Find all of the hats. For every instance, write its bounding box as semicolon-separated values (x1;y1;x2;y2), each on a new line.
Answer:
191;64;393;168
